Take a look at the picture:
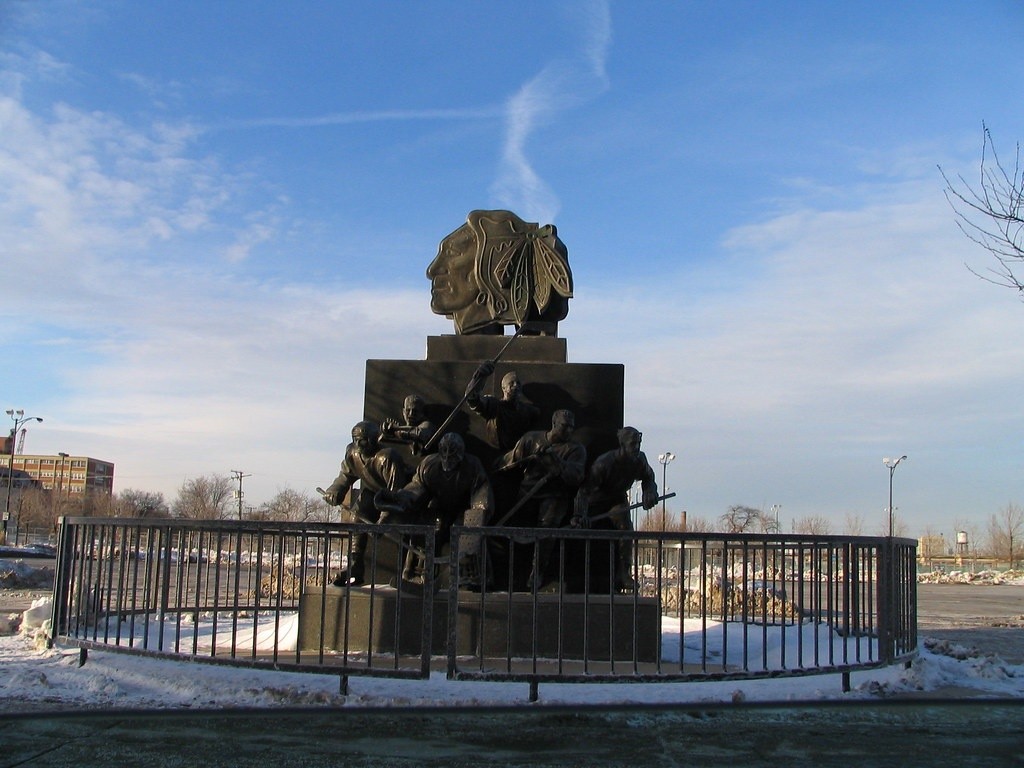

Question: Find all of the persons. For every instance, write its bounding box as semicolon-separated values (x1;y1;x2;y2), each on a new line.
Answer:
323;360;659;593
427;210;574;335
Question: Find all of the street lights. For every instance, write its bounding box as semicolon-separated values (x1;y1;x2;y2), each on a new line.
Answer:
3;408;44;541
771;503;783;531
883;506;899;537
882;454;909;537
658;451;676;533
